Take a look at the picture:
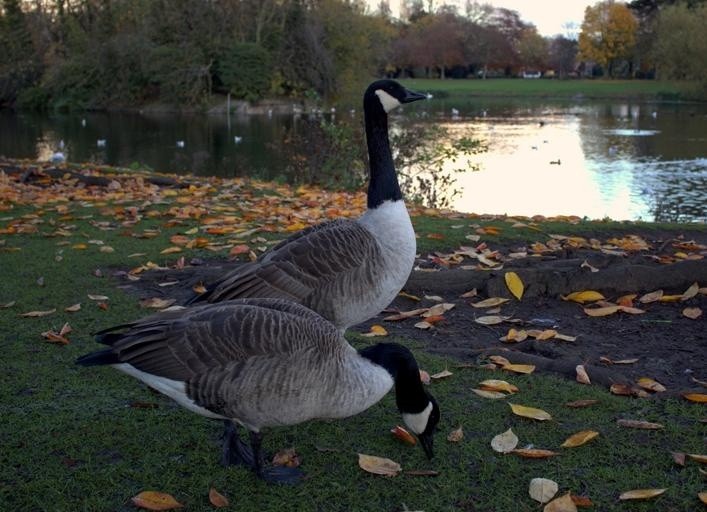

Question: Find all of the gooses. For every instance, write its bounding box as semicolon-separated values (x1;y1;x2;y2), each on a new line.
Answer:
73;297;441;483
182;79;429;340
